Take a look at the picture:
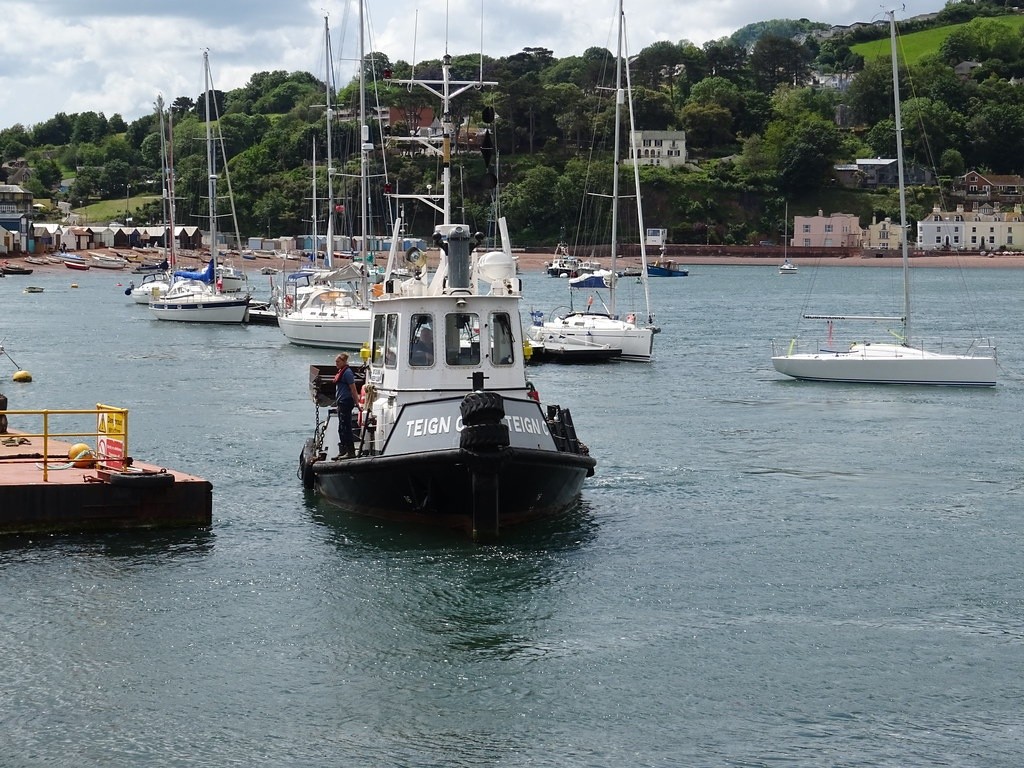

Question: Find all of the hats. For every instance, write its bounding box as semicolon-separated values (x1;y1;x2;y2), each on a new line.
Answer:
338;352;349;362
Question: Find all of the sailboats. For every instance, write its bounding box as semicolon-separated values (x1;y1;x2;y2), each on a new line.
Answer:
128;0;689;539
779;202;798;274
772;9;1003;387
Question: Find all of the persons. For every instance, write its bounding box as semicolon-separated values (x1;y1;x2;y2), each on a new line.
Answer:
331;354;363;461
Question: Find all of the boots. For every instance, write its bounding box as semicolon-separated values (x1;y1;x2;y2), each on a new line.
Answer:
338;445;355;459
331;443;347;460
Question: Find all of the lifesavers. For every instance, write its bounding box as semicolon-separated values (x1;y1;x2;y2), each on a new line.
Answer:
357;386;365;427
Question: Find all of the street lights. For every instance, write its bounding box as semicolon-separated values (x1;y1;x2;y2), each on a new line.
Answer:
126;184;131;227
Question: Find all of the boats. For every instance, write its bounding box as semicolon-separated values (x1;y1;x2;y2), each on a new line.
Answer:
0;247;127;294
